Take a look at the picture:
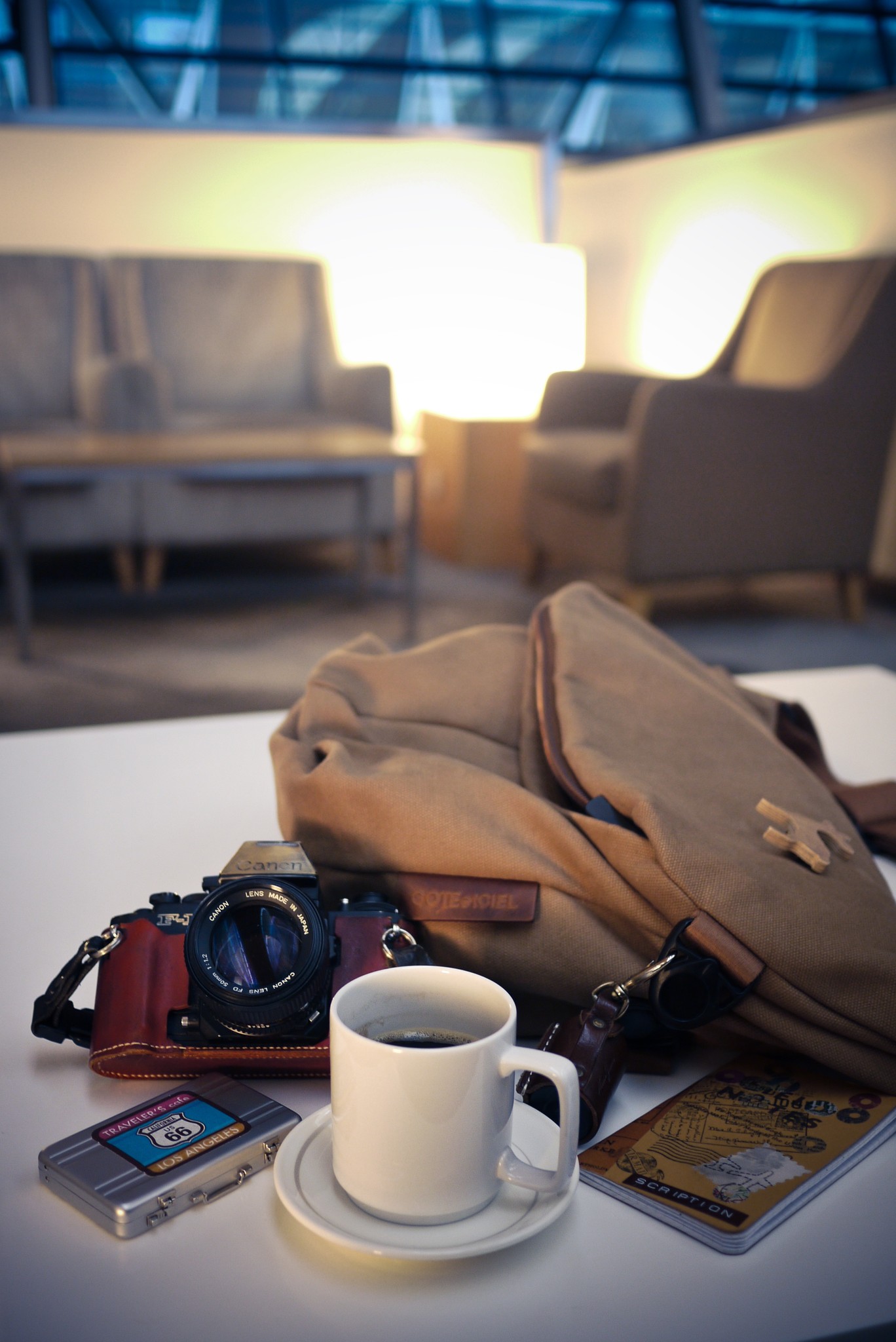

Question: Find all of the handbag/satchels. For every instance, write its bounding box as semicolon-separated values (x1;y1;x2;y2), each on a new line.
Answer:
270;572;896;1097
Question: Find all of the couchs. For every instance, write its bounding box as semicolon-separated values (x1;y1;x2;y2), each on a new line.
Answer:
524;256;896;624
0;250;143;597
93;254;404;598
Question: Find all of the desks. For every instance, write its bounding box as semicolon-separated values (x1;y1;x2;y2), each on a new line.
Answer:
0;662;896;1342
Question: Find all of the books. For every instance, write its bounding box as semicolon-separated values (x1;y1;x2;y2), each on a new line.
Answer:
578;1054;896;1255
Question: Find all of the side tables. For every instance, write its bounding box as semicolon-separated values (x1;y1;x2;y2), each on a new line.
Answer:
416;410;533;574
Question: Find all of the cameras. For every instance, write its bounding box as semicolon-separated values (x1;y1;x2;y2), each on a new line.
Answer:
89;839;422;1084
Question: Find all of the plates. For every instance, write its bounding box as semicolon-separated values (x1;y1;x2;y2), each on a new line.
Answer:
273;1098;581;1259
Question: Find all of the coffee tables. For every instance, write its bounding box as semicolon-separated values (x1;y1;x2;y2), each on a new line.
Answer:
0;425;426;665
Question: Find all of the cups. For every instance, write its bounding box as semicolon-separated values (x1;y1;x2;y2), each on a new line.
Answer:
329;965;580;1227
